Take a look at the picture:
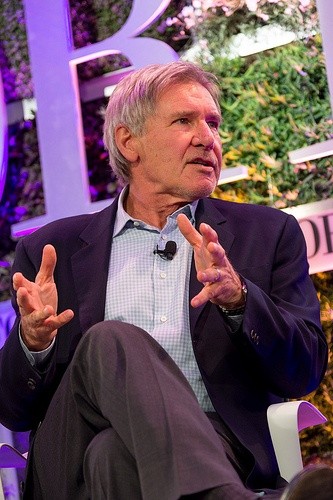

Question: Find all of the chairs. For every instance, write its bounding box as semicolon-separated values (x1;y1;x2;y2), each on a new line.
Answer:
0;400;327;500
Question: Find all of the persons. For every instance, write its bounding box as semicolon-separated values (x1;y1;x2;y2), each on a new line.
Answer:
0;62;329;500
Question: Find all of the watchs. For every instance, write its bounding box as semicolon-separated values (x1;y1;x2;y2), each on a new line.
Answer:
217;281;247;315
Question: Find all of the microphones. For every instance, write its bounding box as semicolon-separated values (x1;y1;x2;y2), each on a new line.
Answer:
163;240;176;260
